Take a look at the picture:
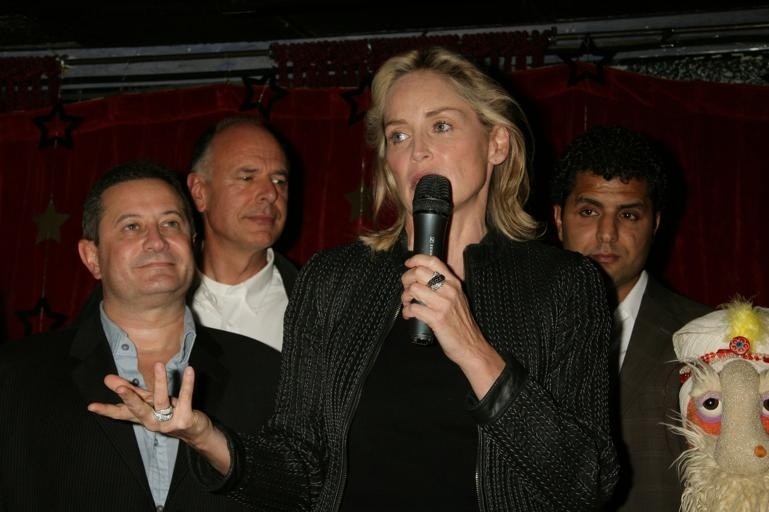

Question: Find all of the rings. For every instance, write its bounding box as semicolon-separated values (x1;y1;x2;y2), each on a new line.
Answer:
426;272;445;290
154;406;173;421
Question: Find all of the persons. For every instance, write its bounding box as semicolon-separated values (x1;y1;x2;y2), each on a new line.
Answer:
86;47;628;510
185;116;306;353
0;159;281;512
552;122;715;511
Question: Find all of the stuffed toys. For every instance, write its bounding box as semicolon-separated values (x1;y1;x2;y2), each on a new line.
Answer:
657;293;769;512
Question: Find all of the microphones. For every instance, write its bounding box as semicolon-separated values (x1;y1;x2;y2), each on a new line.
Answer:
410;173;455;346
